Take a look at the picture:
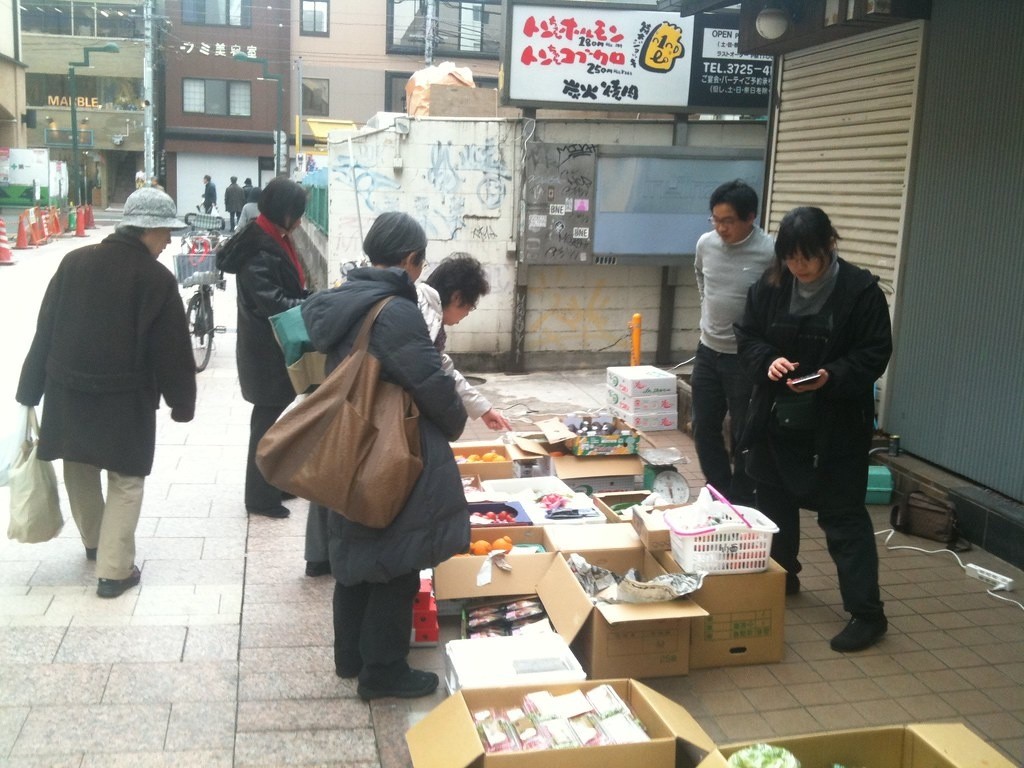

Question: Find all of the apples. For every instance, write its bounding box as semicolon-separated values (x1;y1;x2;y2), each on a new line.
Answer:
472;510;516;524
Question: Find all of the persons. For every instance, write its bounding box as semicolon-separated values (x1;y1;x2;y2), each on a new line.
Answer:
242;178;253;198
732;207;893;653
235;187;263;233
151;177;165;191
202;175;216;214
16;188;195;599
300;211;472;699
692;178;776;506
224;176;245;232
304;253;511;578
215;176;313;518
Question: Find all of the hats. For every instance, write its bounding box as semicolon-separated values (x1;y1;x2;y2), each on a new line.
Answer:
117;187;188;230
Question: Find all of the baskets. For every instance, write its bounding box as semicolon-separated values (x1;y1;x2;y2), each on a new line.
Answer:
176;254;220;284
662;482;779;575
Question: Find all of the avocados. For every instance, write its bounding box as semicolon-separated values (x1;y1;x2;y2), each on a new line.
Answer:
568;420;622;437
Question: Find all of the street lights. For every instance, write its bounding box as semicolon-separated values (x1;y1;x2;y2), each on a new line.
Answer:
69;41;121;208
234;52;285;181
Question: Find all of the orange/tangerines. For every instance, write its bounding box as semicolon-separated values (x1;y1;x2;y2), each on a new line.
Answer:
454;452;506;462
471;535;513;556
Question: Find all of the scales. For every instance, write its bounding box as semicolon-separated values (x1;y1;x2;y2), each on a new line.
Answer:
635;446;691;505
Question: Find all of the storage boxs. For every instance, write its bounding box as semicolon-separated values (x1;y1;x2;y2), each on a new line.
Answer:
405;365;1016;768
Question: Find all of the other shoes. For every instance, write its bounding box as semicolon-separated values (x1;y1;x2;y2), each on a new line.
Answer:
306;561;330;576
245;504;290;518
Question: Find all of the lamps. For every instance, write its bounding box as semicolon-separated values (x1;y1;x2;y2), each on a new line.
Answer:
21;109;37;129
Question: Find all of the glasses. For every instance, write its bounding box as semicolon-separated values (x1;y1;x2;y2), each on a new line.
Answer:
708;214;743;226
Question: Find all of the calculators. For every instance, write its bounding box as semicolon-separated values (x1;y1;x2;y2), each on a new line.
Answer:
791;373;821;385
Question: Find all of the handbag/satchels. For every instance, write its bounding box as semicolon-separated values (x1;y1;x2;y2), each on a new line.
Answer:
8;406;62;543
256;295;423;530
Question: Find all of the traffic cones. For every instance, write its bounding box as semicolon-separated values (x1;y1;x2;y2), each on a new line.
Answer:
0;218;18;265
73;207;91;238
28;207;61;246
11;216;33;250
84;204;100;230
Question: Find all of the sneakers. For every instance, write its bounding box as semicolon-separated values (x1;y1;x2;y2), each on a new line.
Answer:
86;547;98;561
831;601;887;651
97;565;140;598
335;666;361;677
785;578;801;594
358;669;438;698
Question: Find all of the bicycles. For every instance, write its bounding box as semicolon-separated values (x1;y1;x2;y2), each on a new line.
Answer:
174;212;226;373
